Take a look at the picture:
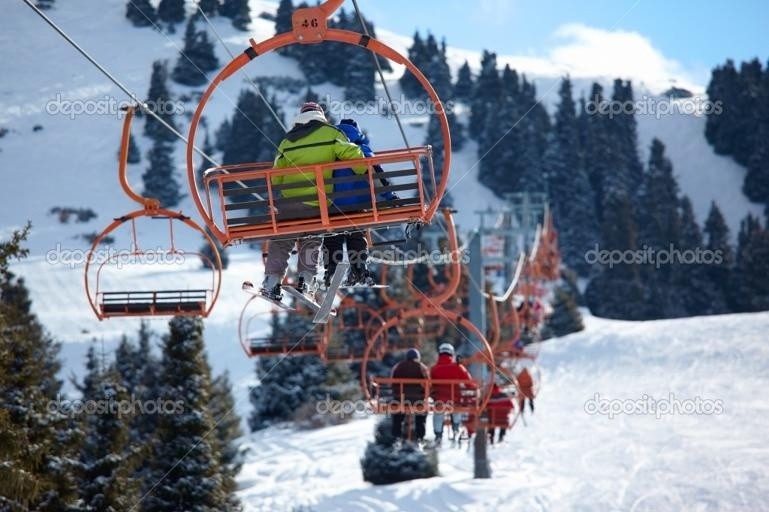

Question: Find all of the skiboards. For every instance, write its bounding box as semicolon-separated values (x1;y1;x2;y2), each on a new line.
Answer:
243;282;337;317
313;263;389;323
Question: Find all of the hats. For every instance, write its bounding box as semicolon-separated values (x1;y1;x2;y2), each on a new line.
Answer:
300;100;322;114
406;349;422;359
438;344;455;356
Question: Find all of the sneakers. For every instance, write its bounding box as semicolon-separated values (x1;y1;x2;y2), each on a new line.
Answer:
323;269;376;285
290;278;337;316
257;276;282;303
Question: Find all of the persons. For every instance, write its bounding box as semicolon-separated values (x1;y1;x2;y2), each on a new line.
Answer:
261;104;369;301
322;118;400;289
390;343;513;448
516;368;534;413
